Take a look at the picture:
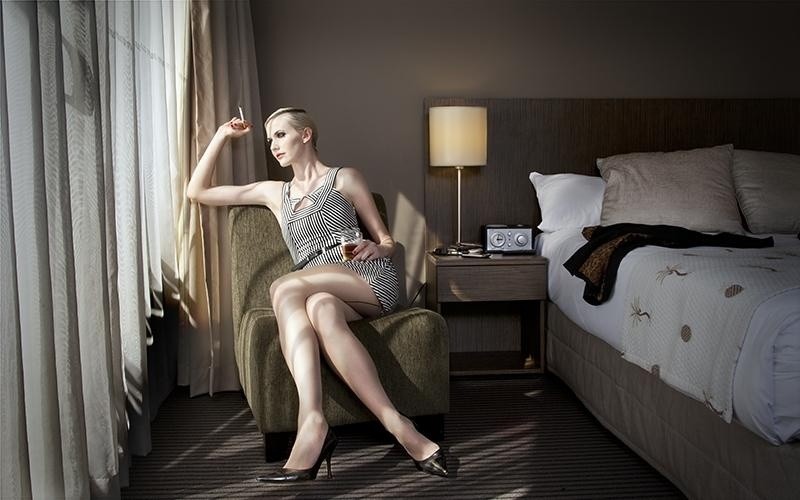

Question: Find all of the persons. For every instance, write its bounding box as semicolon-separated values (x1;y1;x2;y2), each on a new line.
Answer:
187;107;448;482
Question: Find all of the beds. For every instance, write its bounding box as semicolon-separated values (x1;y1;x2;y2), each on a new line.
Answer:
533;230;798;500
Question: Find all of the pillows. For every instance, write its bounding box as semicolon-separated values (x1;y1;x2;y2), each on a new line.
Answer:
527;166;606;235
591;140;749;239
729;144;800;235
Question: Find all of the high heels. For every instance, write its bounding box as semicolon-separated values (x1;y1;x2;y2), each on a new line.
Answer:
396;415;451;480
254;423;340;483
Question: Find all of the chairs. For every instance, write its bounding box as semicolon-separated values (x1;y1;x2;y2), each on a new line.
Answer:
225;188;454;465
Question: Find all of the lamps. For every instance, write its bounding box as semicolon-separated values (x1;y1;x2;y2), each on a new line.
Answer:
426;104;489;258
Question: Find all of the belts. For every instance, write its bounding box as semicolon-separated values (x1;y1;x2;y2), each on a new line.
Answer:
290;242;342;272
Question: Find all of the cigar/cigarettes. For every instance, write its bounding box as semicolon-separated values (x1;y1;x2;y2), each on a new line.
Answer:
238;105;246;129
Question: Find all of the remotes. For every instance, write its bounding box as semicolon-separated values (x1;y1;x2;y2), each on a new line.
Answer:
461;254;491;258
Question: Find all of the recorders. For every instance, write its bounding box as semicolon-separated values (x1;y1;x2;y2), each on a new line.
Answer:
478;224;537;255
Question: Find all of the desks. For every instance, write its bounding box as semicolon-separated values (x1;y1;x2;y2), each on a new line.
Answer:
425;247;554;390
